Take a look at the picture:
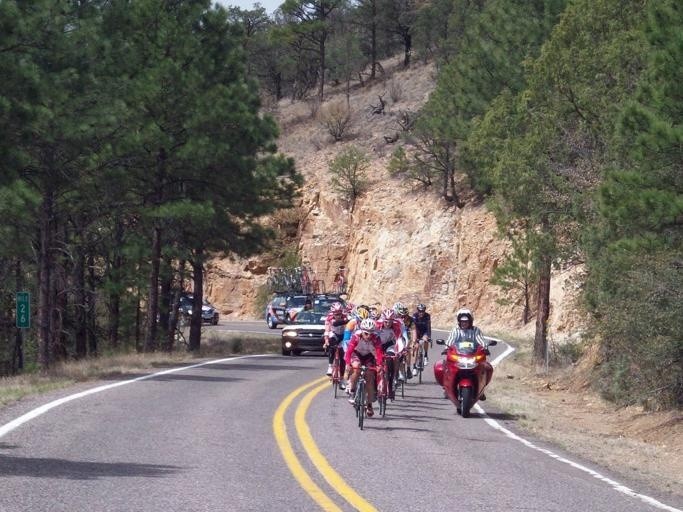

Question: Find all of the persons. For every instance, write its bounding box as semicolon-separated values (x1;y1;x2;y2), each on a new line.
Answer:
442;308;493;401
296;298;319;320
326;297;431;416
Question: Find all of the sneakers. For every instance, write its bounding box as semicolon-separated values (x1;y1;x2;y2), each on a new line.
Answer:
366;402;373;416
398;371;404;381
339;382;346;389
423;356;428;365
348;390;355;404
412;368;418;376
406;368;412;378
327;366;333;376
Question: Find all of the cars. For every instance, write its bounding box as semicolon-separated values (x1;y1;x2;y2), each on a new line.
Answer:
156;290;219;325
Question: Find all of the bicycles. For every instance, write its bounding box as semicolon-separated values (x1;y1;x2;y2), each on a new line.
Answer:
323;331;432;430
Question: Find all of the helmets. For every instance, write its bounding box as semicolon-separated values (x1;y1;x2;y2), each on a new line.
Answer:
330;302;409;335
417;304;426;312
457;309;472;328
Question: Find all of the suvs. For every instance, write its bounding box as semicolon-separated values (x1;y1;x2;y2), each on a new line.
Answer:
266;295;355;358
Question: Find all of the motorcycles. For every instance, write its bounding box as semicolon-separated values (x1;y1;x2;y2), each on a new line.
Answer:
434;339;498;418
